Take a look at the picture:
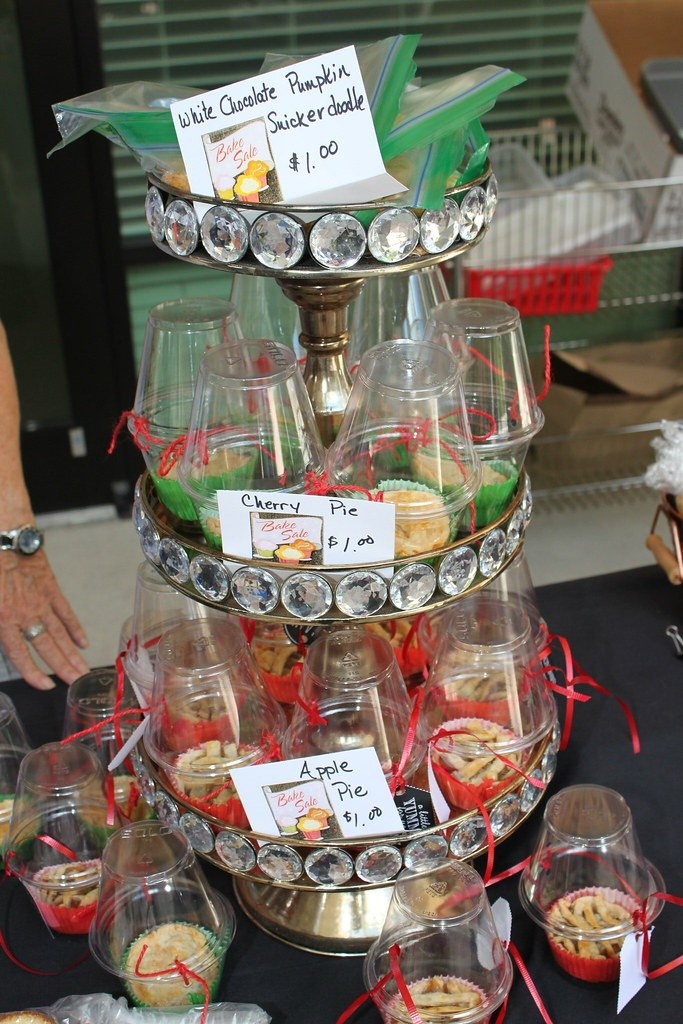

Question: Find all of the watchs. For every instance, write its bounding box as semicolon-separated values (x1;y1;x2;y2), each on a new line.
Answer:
0;523;45;556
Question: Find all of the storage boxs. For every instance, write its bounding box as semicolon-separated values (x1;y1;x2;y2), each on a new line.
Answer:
563;0;683;240
530;334;683;477
463;165;636;289
487;144;553;215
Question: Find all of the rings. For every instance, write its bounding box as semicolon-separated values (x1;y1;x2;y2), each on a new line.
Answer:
26;623;45;640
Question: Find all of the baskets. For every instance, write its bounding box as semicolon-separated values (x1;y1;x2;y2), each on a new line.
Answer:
439;242;615;315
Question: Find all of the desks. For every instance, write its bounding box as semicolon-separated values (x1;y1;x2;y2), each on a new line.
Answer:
1;567;683;1024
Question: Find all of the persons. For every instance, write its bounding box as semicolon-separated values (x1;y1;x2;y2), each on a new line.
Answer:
0;321;92;691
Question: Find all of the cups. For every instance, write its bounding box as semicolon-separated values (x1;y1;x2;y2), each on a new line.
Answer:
0;267;665;1024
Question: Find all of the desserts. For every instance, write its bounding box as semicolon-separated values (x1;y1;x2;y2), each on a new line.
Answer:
0;404;651;1024
